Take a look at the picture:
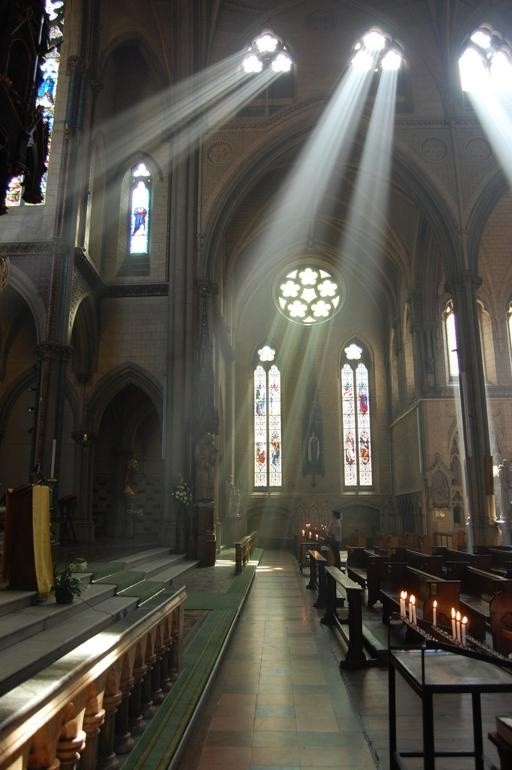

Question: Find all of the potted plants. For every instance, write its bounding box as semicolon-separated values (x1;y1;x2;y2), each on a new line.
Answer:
54;568;88;605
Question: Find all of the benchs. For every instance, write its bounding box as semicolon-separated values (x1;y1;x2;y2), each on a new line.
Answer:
346;543;512;669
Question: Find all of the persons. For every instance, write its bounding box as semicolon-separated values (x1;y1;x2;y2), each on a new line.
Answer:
326;510;342;569
